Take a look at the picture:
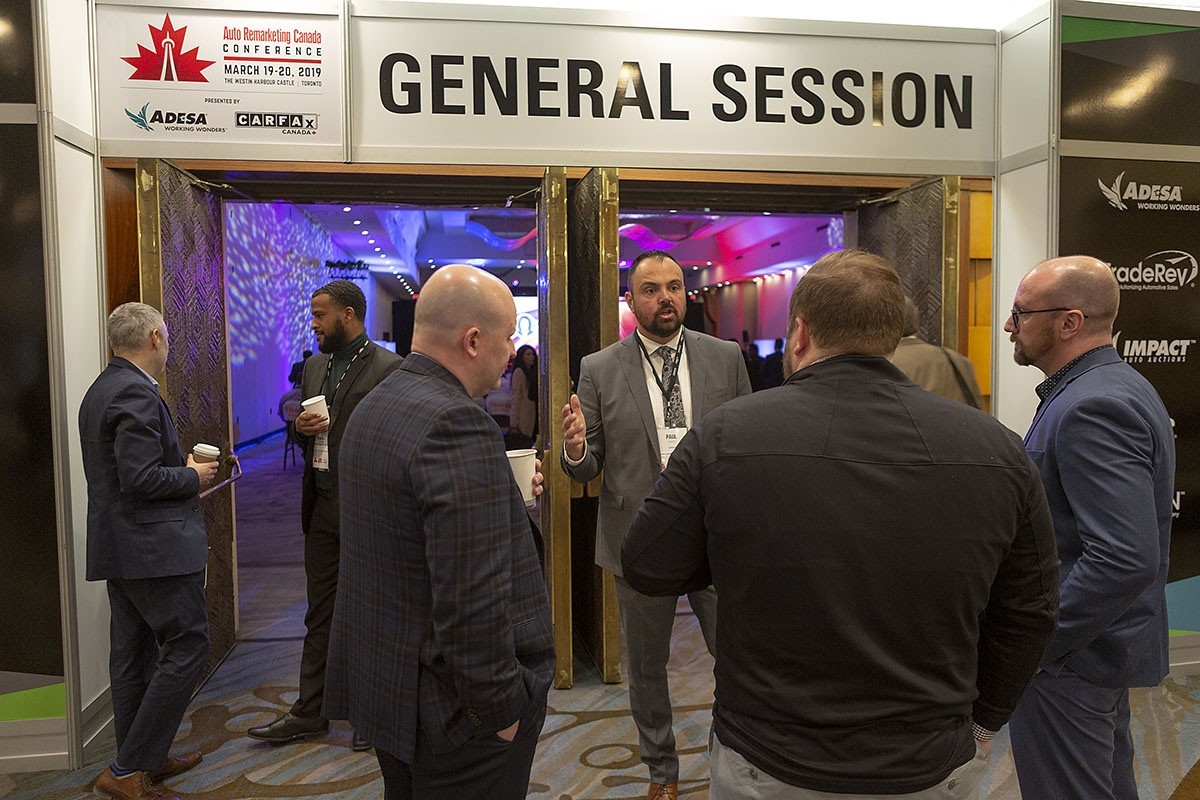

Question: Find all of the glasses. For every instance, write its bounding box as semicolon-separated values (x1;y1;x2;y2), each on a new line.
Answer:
1010;308;1088;328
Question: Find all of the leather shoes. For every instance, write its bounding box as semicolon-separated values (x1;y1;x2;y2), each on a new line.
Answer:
246;712;324;741
648;781;678;800
352;731;372;751
147;752;203;783
93;762;179;800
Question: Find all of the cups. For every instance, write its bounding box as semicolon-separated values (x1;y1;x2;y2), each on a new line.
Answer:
193;443;220;463
506;449;537;507
300;395;330;427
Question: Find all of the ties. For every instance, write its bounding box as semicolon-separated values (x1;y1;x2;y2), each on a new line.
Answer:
653;345;687;429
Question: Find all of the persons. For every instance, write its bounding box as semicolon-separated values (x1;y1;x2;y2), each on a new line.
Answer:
561;249;1056;800
1008;255;1175;800
321;264;556;800
248;280;407;751
79;301;220;800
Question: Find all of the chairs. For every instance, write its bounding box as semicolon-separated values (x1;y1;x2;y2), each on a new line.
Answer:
283;401;300;470
473;390;512;433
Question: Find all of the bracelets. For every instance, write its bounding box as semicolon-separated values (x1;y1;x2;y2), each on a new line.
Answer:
972;722;996;742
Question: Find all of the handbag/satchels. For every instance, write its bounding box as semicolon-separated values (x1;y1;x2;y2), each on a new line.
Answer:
501;427;531;450
